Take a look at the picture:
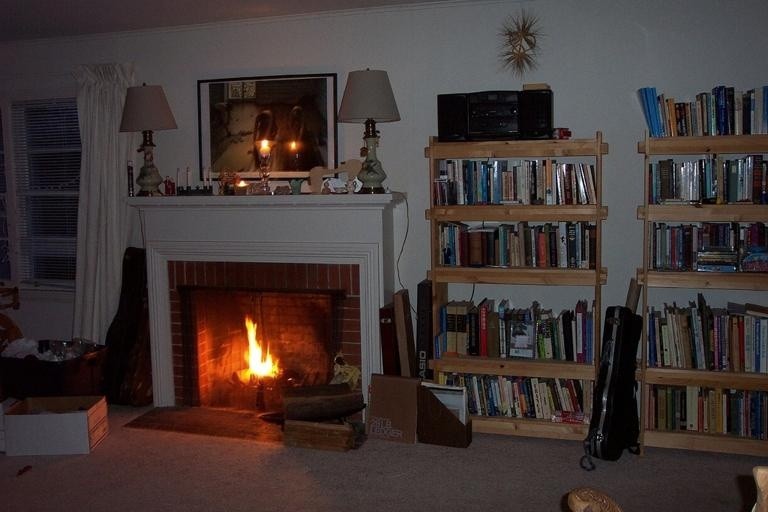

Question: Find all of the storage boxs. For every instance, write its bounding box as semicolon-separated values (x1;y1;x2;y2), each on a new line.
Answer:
4;393;109;460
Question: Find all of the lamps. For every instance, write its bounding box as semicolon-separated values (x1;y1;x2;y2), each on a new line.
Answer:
116;77;177;196
252;135;274;194
335;64;401;194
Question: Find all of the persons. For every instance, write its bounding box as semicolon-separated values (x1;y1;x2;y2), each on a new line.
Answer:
215;99;264;172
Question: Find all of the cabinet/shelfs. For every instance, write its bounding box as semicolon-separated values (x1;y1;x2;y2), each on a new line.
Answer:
638;133;768;463
425;130;605;444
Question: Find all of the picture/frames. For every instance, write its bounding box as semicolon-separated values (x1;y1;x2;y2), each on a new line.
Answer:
196;72;339;182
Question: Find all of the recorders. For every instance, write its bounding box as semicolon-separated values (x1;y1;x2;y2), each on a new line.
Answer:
438;90;553;140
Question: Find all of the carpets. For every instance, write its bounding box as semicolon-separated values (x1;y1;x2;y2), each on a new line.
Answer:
122;406;283;446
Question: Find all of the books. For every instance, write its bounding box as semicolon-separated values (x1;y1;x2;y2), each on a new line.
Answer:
638;86;767;205
434;160;596;208
439;372;596;426
647;293;767;373
647;221;767;273
434;298;596;366
645;383;766;443
439;220;596;270
